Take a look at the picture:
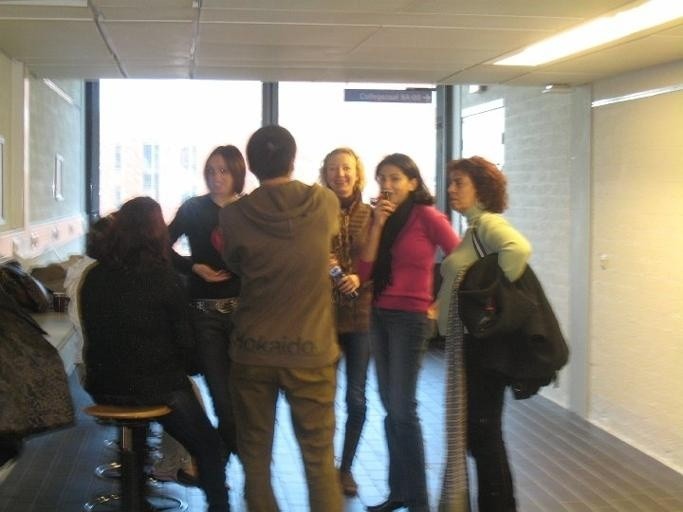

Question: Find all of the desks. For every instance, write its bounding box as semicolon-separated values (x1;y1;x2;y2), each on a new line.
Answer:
0;261;81;353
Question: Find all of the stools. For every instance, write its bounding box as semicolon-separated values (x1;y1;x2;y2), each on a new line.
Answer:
83;402;182;512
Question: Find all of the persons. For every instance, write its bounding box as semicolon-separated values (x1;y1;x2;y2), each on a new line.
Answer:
210;122;347;512
74;193;232;512
434;152;536;512
62;210;207;489
315;145;374;500
352;151;464;512
165;141;248;462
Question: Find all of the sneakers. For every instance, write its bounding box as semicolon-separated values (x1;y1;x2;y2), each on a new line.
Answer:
340;471;357;495
177;468;230;490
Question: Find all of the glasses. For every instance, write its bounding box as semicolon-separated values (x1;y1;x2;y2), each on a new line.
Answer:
207;167;226;174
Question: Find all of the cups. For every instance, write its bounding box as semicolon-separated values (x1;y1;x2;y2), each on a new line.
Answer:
52;292;69;311
381;191;392;203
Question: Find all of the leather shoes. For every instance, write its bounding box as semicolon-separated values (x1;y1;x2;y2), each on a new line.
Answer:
368;500;408;512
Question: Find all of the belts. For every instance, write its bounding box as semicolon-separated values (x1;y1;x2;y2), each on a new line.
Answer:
187;297;240;314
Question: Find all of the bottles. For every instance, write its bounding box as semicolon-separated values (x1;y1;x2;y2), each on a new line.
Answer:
328;260;359;298
